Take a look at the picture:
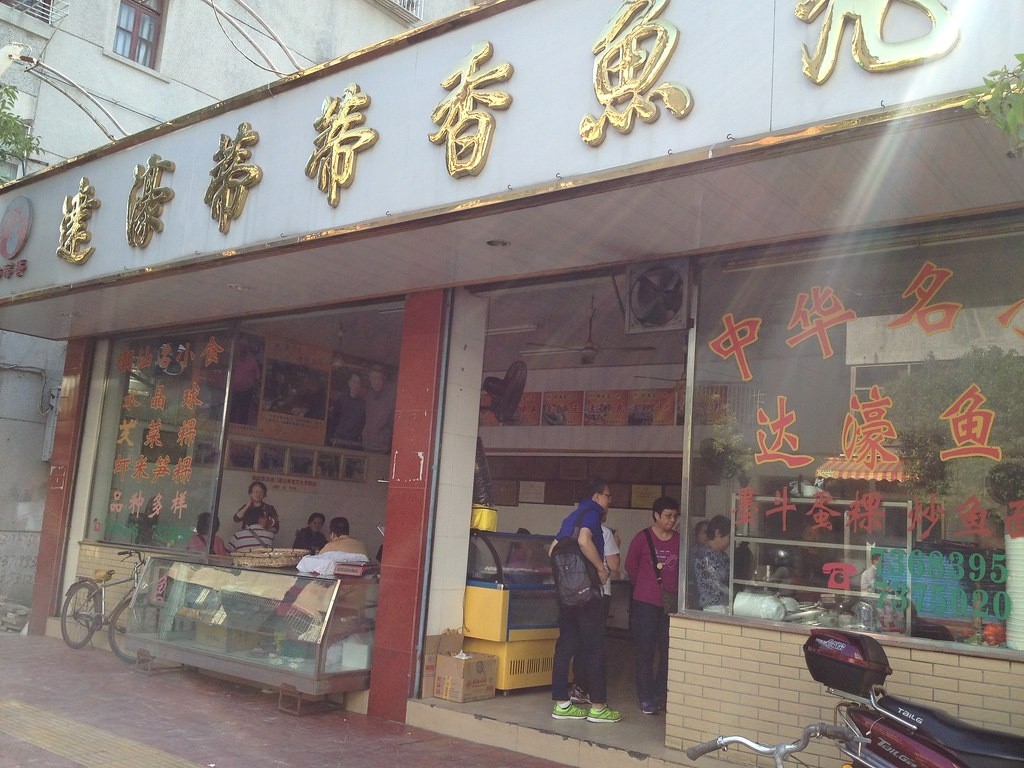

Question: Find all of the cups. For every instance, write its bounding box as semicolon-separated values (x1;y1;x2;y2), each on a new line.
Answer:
839;615;853;627
803;486;817;498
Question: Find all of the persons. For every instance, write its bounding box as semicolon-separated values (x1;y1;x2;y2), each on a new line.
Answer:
861;553;895;593
328;366;394;454
375;543;383;563
233;482;279;534
224;507;272;553
293;512;328;556
802;524;830;586
547;476;621;723
316;517;369;560
687;518;708;610
571;496;621;704
187;512;225;555
693;515;736;607
209;336;327;436
624;495;681;714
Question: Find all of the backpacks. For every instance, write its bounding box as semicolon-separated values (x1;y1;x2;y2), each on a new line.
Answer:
550;508;603;612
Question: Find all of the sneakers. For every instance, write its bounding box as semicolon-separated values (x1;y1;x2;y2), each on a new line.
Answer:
551;701;589;720
587;703;623;722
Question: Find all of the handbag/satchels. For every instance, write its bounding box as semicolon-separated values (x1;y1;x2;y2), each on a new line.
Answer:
661;588;678;614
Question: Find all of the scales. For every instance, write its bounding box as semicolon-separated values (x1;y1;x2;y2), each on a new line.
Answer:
334;561;378;577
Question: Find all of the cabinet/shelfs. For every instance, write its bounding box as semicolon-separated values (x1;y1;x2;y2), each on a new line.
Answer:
730;493;913;637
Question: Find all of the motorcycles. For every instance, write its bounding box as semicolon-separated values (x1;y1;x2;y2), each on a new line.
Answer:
802;628;1024;767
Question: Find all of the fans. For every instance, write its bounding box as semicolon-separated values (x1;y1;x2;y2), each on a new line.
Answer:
701;437;752;488
484;360;529;423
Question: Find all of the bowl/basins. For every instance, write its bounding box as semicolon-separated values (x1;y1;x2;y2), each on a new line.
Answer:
1004;533;1024;651
733;591;786;621
817;615;837;627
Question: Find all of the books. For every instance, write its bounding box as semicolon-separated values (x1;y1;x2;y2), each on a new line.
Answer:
377;525;384;534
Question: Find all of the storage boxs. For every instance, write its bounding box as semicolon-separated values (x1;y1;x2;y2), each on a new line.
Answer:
195;612;260;650
802;628;893;699
420;633;496;703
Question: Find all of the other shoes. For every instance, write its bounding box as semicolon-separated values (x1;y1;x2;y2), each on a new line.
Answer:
569;684;592;703
641;700;655;713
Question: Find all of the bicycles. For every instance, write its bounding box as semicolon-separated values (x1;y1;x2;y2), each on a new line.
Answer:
61;549;158;664
685;721;872;768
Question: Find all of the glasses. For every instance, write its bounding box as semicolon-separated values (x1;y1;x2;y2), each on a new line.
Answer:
598;491;612;504
